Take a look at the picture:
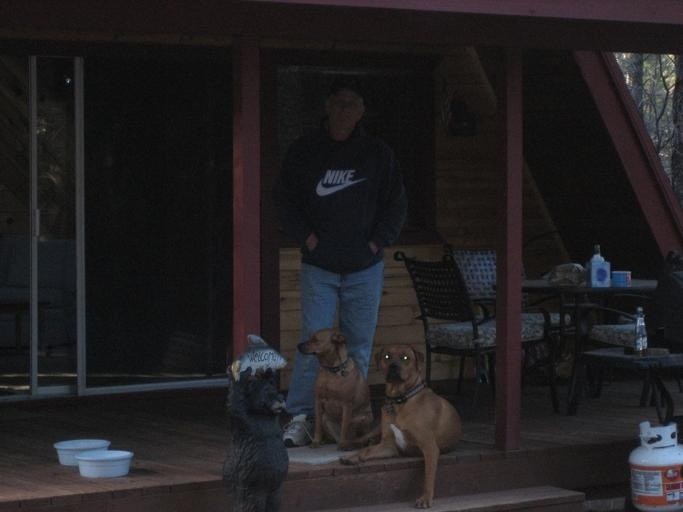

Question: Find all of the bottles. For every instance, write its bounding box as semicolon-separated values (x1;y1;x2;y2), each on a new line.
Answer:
634;307;648;354
589;244;605;262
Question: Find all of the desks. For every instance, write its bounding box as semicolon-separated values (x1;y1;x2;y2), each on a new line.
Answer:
520;278;658;415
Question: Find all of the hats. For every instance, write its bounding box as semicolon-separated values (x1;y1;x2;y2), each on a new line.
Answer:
327;74;364;97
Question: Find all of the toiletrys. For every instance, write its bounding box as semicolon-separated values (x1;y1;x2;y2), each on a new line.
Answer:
586;253;611;289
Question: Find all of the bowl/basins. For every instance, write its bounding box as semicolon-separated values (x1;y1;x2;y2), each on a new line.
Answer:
75;449;134;479
53;439;111;467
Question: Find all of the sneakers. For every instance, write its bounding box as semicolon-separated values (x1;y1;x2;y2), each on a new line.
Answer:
282;413;314;448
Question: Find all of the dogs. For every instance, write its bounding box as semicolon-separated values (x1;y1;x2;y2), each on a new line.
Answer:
339;343;463;510
295;325;374;453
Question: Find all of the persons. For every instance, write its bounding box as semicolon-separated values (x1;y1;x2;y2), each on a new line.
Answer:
272;71;407;446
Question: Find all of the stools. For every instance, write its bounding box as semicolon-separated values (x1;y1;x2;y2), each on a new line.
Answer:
564;345;683;424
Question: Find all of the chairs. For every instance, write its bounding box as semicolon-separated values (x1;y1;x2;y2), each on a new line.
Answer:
394;250;558;416
444;243;572;388
